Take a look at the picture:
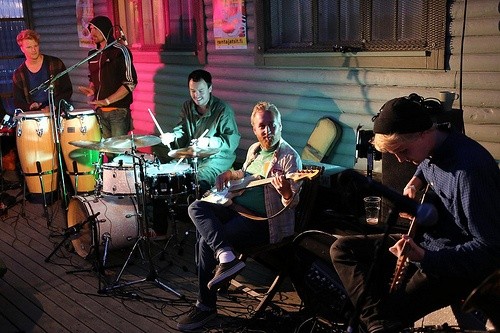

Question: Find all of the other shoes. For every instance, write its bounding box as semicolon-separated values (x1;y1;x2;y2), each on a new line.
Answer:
144;228;166;240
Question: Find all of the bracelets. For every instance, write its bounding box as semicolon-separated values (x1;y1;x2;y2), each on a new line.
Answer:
105;99;110;105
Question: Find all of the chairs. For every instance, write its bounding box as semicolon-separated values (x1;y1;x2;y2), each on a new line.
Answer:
217;179;316;328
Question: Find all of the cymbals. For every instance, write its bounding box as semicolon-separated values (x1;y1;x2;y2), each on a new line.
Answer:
112;151;155;164
68;134;162;154
167;147;220;159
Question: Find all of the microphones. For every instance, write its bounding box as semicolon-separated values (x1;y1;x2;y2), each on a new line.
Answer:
118;26;128;46
0;114;10;129
63;100;75;112
64;226;80;238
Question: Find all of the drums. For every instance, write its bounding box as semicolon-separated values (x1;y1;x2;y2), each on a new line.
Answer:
67;195;143;259
15;110;58;193
144;163;197;197
59;109;103;193
101;162;152;196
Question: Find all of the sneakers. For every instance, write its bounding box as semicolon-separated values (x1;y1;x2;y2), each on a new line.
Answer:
177;304;217;330
207;256;246;290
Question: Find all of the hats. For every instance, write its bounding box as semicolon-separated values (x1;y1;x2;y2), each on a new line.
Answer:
373;97;433;134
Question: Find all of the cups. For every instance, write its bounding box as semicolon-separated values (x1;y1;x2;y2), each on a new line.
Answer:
364;196;381;225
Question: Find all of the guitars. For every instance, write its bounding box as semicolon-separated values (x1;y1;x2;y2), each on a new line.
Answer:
389;183;431;293
196;165;325;207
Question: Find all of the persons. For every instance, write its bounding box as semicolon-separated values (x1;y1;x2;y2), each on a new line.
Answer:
79;15;137;162
13;30;74;113
330;98;500;333
144;69;241;239
178;103;302;328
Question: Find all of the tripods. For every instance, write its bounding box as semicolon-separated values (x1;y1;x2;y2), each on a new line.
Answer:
44;149;193;300
